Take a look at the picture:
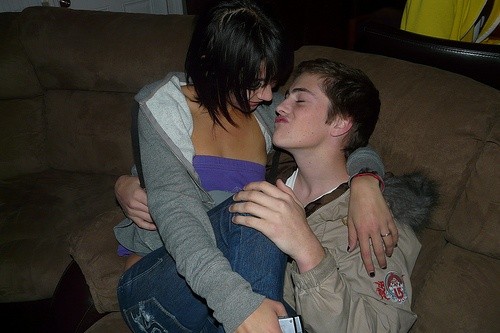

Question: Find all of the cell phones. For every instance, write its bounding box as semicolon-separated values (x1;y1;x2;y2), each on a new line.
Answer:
278;315;304;333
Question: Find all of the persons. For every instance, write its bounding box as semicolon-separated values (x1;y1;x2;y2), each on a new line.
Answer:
46;58;422;333
113;0;400;333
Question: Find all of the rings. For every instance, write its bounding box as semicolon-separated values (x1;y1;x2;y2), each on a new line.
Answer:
381;229;395;241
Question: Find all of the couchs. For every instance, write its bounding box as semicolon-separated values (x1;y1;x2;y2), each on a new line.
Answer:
42;21;499;332
0;6;197;333
